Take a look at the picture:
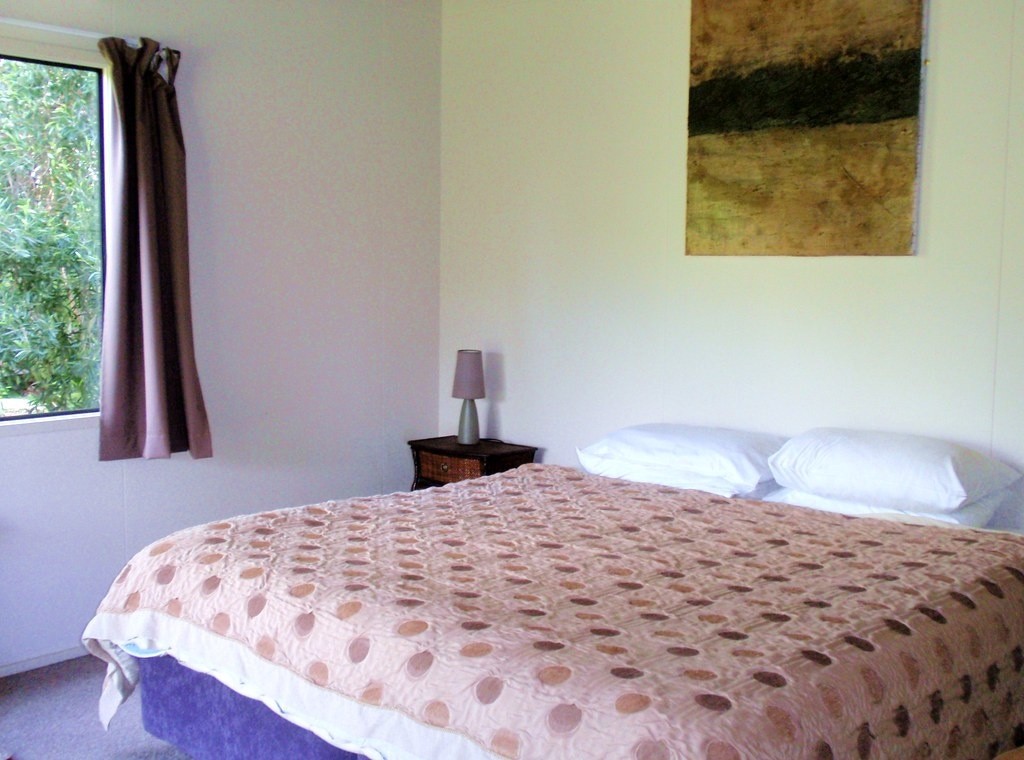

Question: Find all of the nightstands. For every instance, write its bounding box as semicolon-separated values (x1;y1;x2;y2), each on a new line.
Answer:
408;436;539;492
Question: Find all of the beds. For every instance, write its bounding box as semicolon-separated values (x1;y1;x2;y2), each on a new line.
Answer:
82;462;1024;760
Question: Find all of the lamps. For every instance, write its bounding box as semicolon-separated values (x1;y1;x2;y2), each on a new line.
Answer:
452;349;485;444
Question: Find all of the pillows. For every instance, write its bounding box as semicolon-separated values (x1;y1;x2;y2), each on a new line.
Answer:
768;427;1020;512
761;487;1004;527
577;423;788;499
